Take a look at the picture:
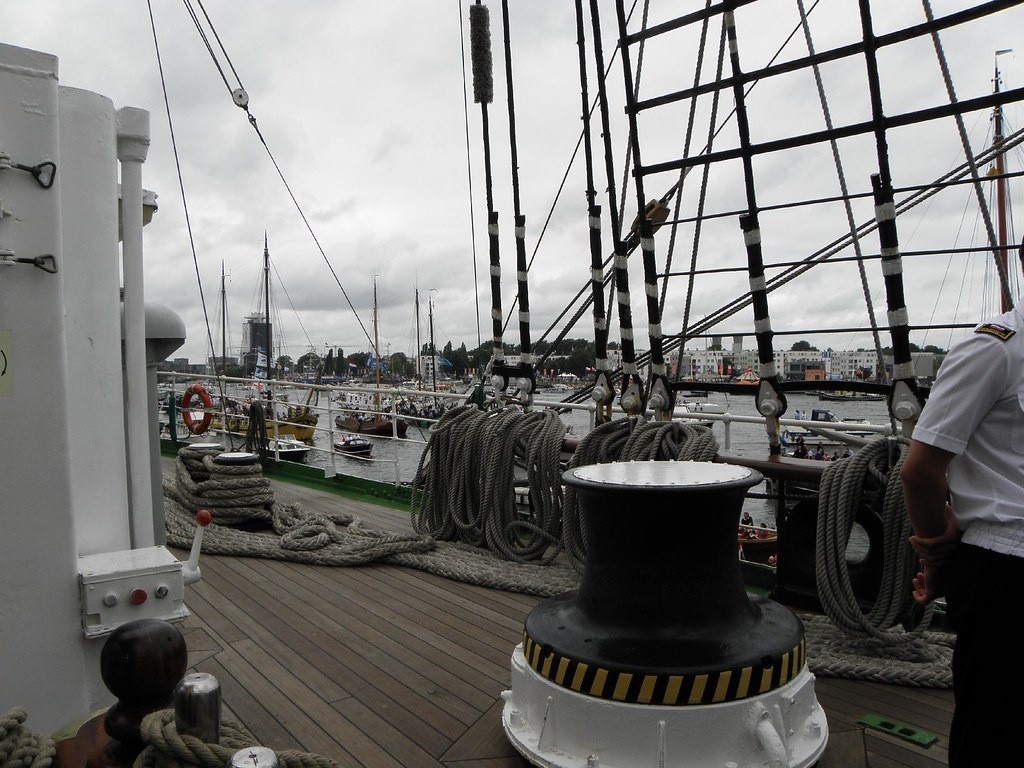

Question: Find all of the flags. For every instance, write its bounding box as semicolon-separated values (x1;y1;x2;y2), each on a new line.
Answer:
251;382;264;391
441;357;452;367
257;352;275;367
255;368;267;378
349;363;357;369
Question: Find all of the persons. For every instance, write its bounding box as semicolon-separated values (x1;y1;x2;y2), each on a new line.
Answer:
695;402;702;411
356;404;392;422
342;434;360;442
794;435;853;461
900;233;1024;768
795;410;808;420
739;512;769;539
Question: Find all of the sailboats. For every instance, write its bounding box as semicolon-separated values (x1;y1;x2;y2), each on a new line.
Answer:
271;334;289;401
654;315;730;431
332;273;456;435
188;230;325;438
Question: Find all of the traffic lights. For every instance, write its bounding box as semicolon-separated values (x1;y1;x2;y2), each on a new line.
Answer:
258;433;311;463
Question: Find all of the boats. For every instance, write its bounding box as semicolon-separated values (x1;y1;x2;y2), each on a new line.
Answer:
334;439;373;457
815;390;887;402
738;521;778;566
780;409;878;457
158;385;188;412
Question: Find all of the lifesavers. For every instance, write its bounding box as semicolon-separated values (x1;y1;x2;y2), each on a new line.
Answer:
182;386;212;435
